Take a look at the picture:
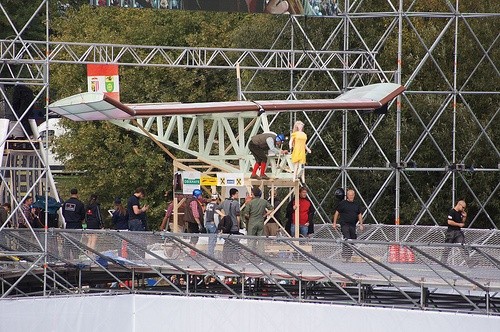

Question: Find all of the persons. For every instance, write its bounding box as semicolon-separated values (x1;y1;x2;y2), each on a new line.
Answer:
62;188;84;260
183;189;204;256
165;275;285;286
214;188;241;264
110;188;150;259
84;194;104;249
245;192;251;230
0;203;11;244
440;201;478;268
264;189;281;236
265;0;304;15
204;192;226;257
249;133;288;180
160;194;187;257
18;195;59;260
289;120;311;183
202;197;224;227
333;190;364;262
244;187;273;262
285;187;314;245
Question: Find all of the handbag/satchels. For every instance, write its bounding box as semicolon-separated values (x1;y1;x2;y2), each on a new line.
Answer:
218;215;234;232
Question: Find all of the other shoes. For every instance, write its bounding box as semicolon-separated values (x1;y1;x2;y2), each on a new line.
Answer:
260;174;271;180
250;174;260;180
468;259;480;268
342;257;353;263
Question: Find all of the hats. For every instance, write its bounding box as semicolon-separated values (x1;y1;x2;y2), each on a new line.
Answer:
193;189;203;198
90;194;98;200
115;198;122;205
276;134;286;143
